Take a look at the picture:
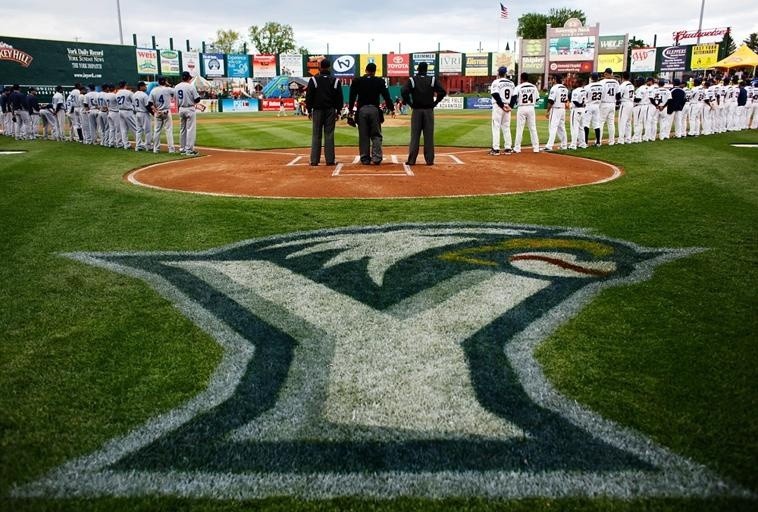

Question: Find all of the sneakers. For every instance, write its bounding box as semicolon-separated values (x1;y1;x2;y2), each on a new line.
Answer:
567;146;576;150
502;149;512;155
374;160;382;165
577;145;586;148
672;135;681;139
540;148;552;151
488;150;501;156
591;143;600;147
310;162;318;166
327;162;335;165
406;162;413;165
688;134;694;136
186;149;199;156
511;150;516;153
616;143;622;144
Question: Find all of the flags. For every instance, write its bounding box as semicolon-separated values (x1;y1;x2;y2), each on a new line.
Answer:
500;2;509;19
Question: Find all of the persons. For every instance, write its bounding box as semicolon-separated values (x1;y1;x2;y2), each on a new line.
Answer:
754;82;758;130
645;77;654;141
714;75;722;133
512;73;540;153
305;60;344;166
52;85;66;141
12;84;30;140
116;80;145;150
722;77;732;133
0;89;4;136
79;87;86;142
67;82;84;143
148;77;175;153
728;78;741;132
737;81;746;130
567;78;586;149
39;102;57;141
4;86;12;136
277;97;287;117
597;68;620;147
670;78;686;139
585;72;601;147
619;71;635;144
132;82;154;152
488;66;515;156
26;87;40;141
348;63;396;165
176;71;200;156
688;77;704;135
632;76;649;143
745;81;754;130
98;84;108;146
652;79;673;143
539;74;568;151
704;82;714;135
83;84;104;146
400;62;447;165
106;83;123;148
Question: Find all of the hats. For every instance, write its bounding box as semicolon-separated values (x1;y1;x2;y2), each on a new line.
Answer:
118;79;126;87
182;71;191;77
158;76;165;82
100;83;106;89
497;67;507;73
137;82;147;87
590;71;598;76
28;88;38;92
634;76;644;82
109;82;115;88
12;84;21;89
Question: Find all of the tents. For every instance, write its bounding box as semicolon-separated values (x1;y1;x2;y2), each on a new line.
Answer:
717;42;758;68
